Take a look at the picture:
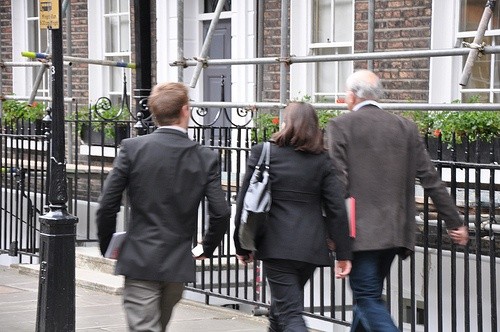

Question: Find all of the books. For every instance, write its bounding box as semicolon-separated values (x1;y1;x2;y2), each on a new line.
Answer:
103;231;130;260
342;197;357;238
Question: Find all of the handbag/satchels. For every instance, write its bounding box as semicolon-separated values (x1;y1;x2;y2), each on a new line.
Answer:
238;141;273;251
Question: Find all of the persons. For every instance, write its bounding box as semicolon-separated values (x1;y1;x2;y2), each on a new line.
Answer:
93;82;232;332
232;102;353;332
320;67;469;332
